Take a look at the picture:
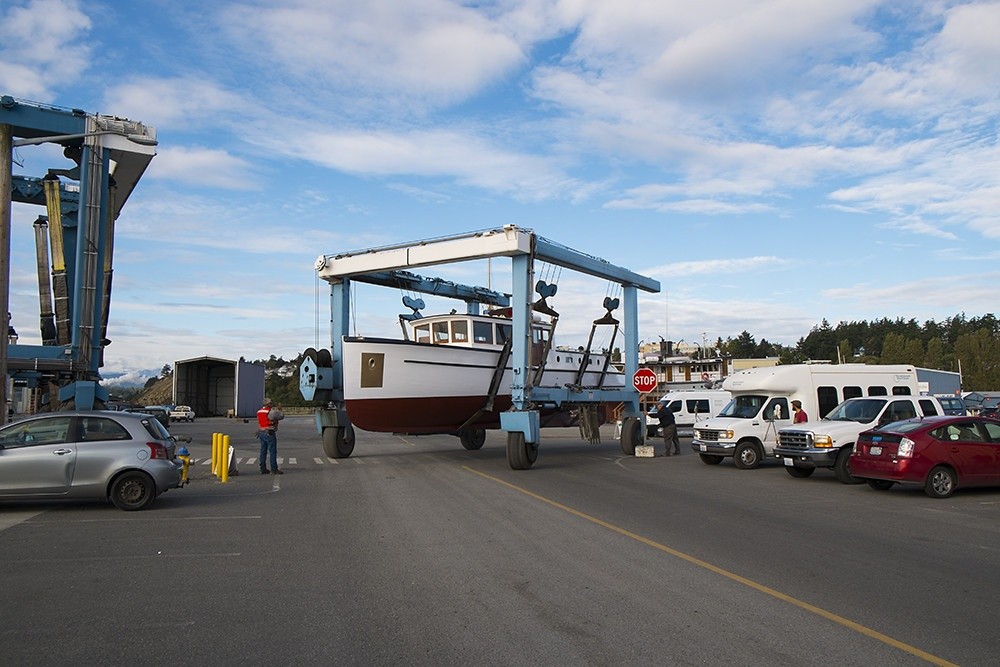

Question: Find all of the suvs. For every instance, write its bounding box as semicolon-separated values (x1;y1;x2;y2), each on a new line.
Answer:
122;408;171;430
771;396;949;485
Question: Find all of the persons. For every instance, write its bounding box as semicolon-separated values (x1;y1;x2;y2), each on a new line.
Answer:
644;402;680;456
791;400;808;424
256;398;285;475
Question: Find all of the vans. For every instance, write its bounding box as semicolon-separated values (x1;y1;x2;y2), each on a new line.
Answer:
691;365;922;470
642;391;736;438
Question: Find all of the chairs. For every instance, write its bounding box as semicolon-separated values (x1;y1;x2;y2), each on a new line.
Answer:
897;408;911;420
84;418;119;441
736;406;757;416
960;430;973;441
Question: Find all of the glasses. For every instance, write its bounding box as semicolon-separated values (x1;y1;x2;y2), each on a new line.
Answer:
269;403;273;404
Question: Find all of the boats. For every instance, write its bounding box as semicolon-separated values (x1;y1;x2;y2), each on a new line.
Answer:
342;308;624;436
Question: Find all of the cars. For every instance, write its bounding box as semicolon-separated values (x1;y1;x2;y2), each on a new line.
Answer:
936;396;966;417
0;410;182;511
849;417;1000;498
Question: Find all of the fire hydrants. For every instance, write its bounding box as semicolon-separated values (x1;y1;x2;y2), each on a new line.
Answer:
175;448;200;484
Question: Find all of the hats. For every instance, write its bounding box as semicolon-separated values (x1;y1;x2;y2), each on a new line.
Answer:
704;373;709;378
261;398;271;405
791;401;801;405
655;402;661;409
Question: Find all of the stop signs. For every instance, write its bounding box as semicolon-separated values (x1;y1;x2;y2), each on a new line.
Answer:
632;368;659;393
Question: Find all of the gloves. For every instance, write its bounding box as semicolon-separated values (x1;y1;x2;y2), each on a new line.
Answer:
254;430;259;439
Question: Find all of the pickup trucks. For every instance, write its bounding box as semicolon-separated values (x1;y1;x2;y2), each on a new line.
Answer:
169;406;196;422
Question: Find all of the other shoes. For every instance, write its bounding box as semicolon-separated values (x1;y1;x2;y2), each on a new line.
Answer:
270;469;284;475
661;453;669;456
260;468;270;474
673;452;679;455
